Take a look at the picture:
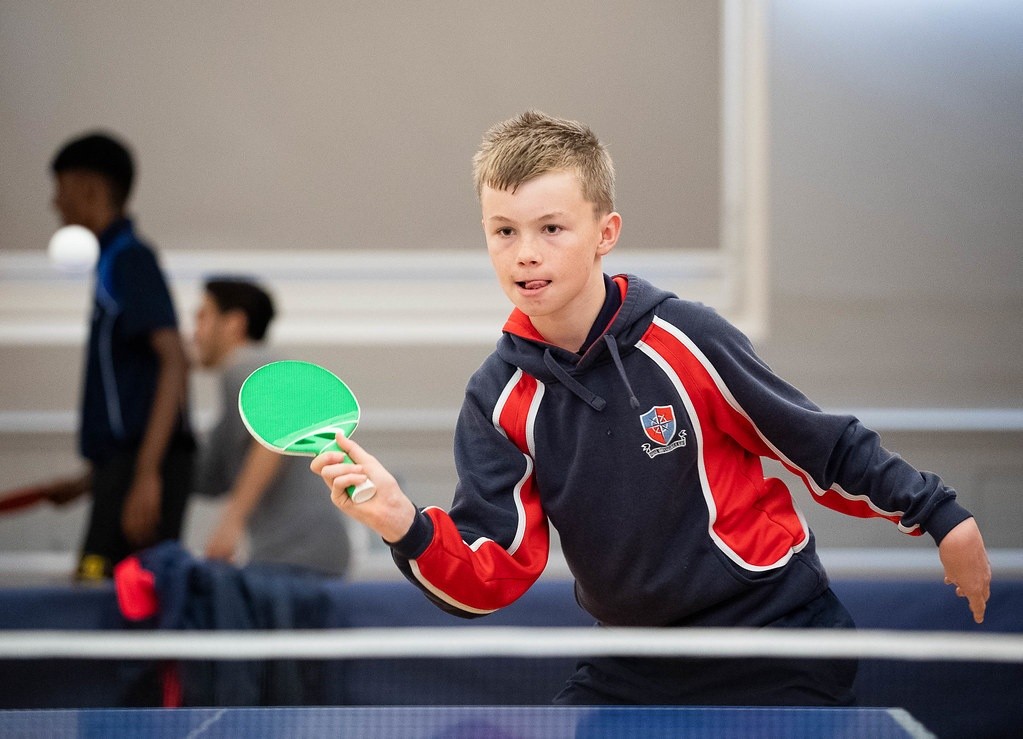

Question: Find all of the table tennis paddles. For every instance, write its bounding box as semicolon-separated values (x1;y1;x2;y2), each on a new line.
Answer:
238;359;378;505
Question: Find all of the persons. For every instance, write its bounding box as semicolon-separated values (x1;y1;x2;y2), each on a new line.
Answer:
309;110;993;705
45;129;354;578
